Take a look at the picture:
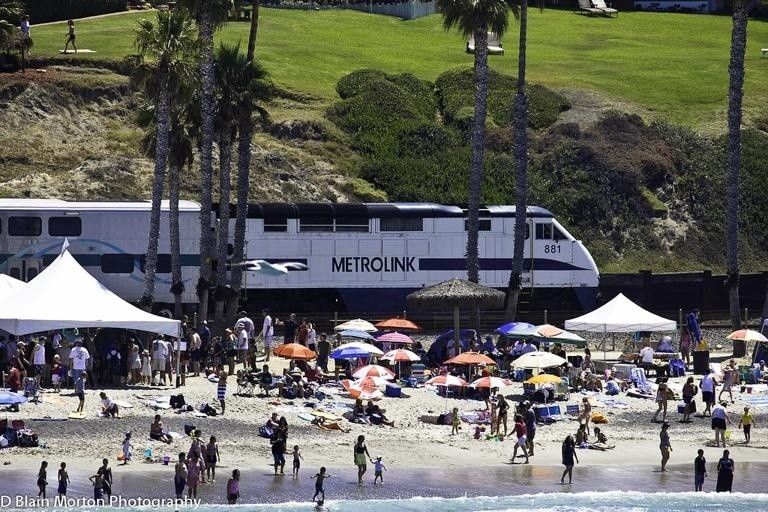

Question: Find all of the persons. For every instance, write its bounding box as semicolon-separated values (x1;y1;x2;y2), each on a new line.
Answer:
63;17;78;53
20;12;31;56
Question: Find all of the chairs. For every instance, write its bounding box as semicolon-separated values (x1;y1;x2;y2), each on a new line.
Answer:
731;362;767;385
437;366;495;400
606;368;650;394
523;376;571;405
236;368;303;398
466;31;477;54
573;0;602;16
639;356;686;376
486;31;506;57
592;0;620;18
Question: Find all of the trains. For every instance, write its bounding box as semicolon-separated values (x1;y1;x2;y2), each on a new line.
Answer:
0;196;601;314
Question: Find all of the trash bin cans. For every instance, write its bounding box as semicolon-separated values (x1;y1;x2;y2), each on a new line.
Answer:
693;351;709;375
733;340;745;357
